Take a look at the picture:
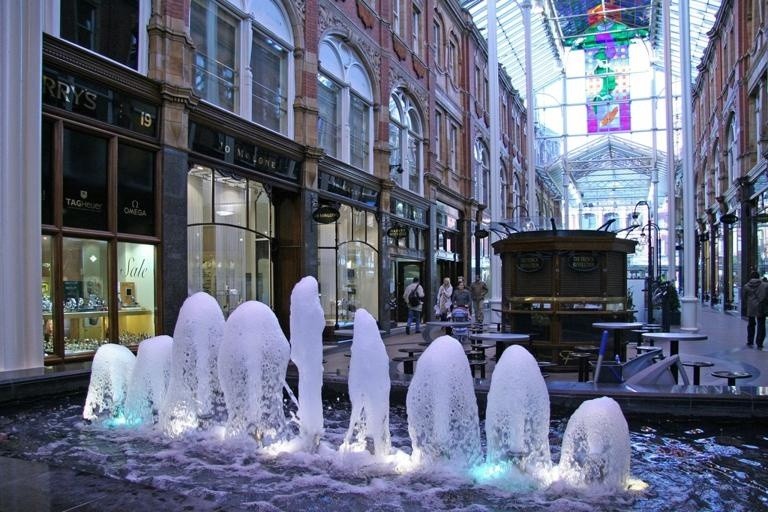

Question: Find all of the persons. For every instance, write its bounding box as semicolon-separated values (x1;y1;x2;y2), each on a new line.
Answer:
742;271;768;347
402;275;488;335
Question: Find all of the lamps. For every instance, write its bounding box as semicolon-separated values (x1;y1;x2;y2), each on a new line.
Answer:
510;205;535;232
631;200;661;280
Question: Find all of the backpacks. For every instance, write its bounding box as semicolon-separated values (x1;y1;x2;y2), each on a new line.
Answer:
408;284;421;307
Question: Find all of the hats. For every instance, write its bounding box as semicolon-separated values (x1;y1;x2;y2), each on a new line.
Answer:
412;277;420;283
443;277;450;283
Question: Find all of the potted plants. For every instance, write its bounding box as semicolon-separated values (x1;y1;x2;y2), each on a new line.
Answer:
670;285;681;324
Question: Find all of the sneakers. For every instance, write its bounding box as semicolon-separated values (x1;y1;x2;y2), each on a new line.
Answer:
746;341;764;350
415;330;421;333
406;325;410;336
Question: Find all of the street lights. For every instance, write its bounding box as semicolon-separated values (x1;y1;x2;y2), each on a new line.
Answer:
632;201;661;346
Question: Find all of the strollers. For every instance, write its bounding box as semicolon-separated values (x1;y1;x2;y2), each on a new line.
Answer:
450;304;471;343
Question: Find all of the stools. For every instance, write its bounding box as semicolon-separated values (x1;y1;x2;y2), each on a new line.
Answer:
569;319;752;387
392;321;559;380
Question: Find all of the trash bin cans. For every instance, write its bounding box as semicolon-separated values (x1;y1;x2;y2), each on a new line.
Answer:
680;297;698;331
